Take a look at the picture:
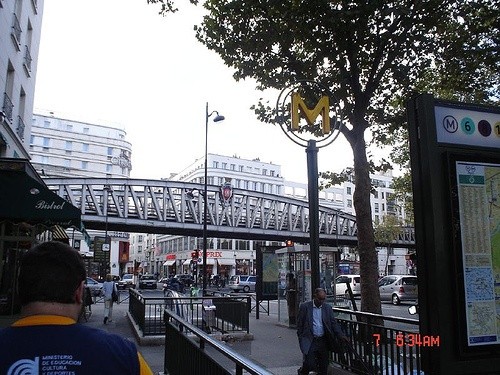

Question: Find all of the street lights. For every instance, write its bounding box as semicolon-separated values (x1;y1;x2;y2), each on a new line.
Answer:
184;101;225;349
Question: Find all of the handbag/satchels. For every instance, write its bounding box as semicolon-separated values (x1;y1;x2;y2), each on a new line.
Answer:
111;282;118;302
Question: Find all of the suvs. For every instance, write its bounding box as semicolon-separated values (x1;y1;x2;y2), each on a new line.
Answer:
227;274;257;293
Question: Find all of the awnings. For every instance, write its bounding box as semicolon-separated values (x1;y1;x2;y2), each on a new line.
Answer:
197;258;216;265
218;259;236;265
183;259;192;265
163;261;175;266
0;170;81;229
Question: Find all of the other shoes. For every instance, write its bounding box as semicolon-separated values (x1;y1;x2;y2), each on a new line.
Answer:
103;317;108;325
108;320;112;322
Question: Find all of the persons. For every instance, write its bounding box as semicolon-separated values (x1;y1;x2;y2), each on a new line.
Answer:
4;248;14;264
101;274;118;324
287;273;295;289
0;240;154;375
296;288;350;375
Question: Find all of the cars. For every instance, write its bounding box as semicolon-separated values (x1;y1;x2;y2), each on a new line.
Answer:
378;274;418;306
121;273;137;289
139;274;158;290
173;273;193;287
84;277;104;297
324;274;361;300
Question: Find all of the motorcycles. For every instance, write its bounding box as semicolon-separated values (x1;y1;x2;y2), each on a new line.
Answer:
162;281;186;295
